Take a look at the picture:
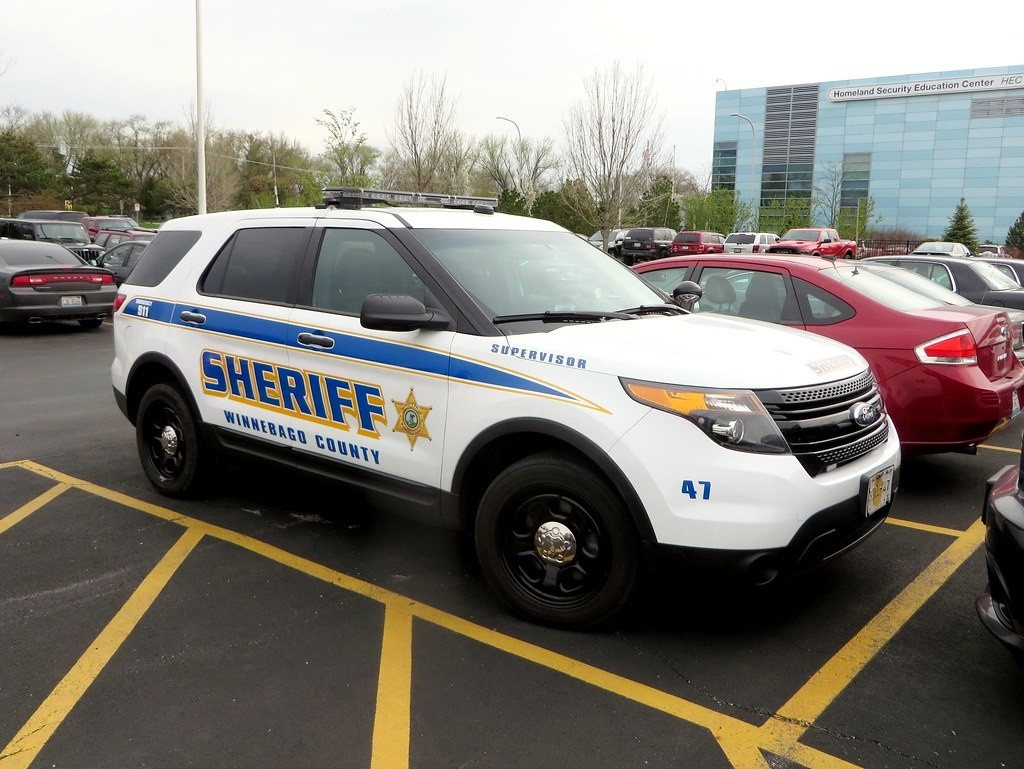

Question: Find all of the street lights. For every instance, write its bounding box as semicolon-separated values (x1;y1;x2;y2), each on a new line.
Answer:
496;116;522;187
730;113;756;231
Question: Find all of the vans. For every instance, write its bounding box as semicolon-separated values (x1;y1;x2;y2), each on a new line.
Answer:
587;229;628;256
19;210;92;225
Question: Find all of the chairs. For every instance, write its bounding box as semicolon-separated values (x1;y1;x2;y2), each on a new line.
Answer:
328;246;388;312
704;275;736;317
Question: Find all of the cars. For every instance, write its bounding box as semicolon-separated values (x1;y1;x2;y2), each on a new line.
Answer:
91;241;151;284
626;252;1024;464
0;236;118;333
976;428;1024;661
910;241;971;257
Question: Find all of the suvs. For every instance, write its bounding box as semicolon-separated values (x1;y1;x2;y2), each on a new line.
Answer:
977;244;1008;258
669;229;726;254
722;232;779;254
94;226;158;249
622;227;680;266
0;217;105;266
80;215;151;242
109;186;902;627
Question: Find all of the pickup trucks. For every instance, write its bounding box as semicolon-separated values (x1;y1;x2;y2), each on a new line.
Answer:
763;228;856;260
